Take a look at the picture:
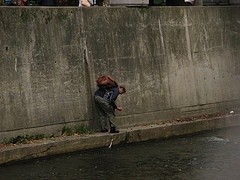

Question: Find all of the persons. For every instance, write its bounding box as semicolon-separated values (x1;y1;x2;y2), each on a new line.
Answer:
94;86;127;133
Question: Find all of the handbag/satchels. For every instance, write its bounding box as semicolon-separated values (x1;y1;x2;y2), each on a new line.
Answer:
81;0;91;7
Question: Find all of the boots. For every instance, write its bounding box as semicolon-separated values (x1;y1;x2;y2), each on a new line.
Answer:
101;128;108;132
110;127;120;133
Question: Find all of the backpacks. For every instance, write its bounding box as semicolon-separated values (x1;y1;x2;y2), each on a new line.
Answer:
96;75;118;92
